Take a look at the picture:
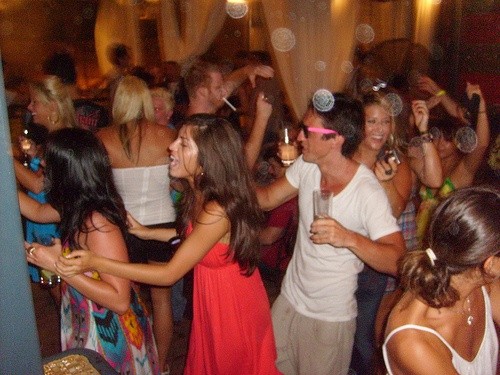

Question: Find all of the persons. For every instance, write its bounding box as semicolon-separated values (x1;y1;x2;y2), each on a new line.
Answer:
107;43;301;286
54;114;282;375
18;127;161;375
348;68;500;375
6;51;111;314
91;75;181;375
381;186;500;375
249;94;405;375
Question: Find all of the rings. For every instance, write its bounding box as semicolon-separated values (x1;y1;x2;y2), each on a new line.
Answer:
27;247;35;255
259;96;264;98
473;89;476;93
385;170;393;175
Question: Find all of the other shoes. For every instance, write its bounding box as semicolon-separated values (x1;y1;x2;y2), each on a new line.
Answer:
161;364;170;375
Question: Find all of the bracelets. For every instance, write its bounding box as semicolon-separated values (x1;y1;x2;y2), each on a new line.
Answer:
478;110;487;114
419;130;433;143
435;90;445;96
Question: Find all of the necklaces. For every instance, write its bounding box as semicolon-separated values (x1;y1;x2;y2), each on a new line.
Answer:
445;295;473;326
351;156;374;170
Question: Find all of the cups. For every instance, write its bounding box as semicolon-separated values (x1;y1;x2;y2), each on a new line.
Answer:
279;128;297;163
313;189;334;222
37;233;55;287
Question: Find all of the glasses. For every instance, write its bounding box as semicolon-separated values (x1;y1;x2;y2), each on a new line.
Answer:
298;123;342;139
